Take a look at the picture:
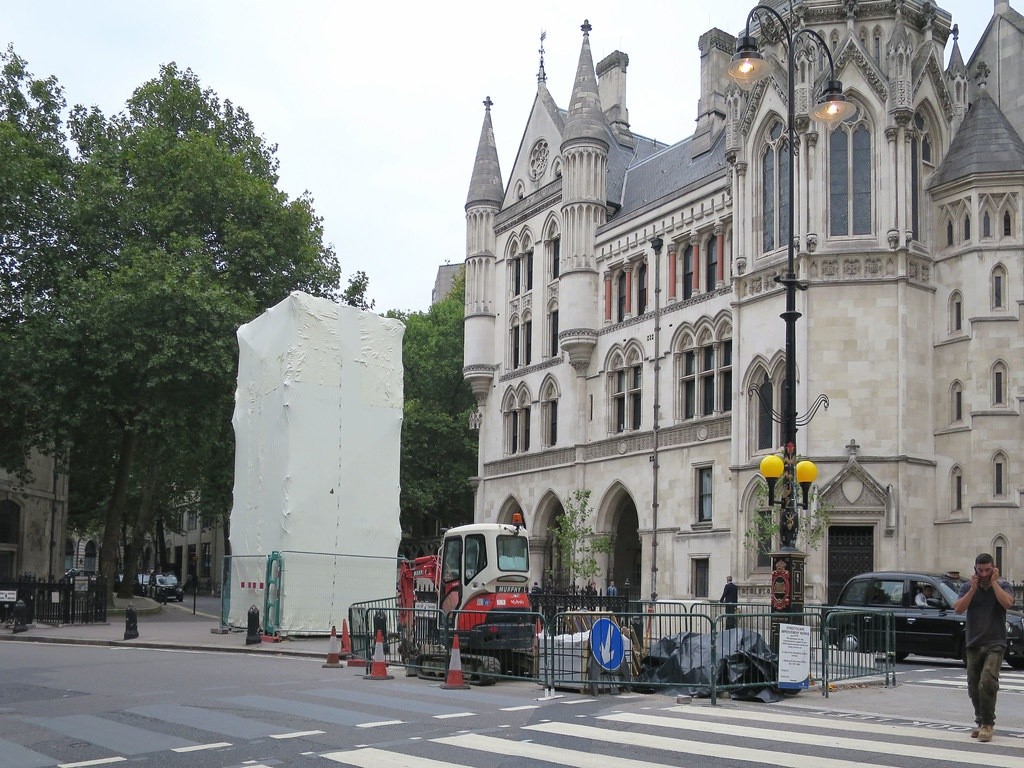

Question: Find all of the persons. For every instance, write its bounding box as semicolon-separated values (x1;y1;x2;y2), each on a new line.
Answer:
915;585;932;607
607;581;617;596
718;576;737;629
590;581;597;595
532;582;539;594
955;553;1014;741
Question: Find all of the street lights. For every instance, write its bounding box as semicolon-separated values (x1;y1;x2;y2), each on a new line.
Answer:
726;3;859;697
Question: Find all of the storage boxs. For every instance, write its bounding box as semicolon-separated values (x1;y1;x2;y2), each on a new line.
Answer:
538;638;632;694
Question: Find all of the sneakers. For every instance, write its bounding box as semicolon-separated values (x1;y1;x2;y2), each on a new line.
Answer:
972;724;981;738
978;723;993;742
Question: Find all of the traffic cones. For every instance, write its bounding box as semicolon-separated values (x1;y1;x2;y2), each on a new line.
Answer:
321;625;344;669
438;633;471;691
362;629;395;681
339;618;352;658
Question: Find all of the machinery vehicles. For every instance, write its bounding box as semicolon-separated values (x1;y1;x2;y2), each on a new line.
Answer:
392;512;544;686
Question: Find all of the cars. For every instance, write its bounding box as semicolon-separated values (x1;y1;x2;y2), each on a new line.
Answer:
115;569;184;603
820;570;1024;672
